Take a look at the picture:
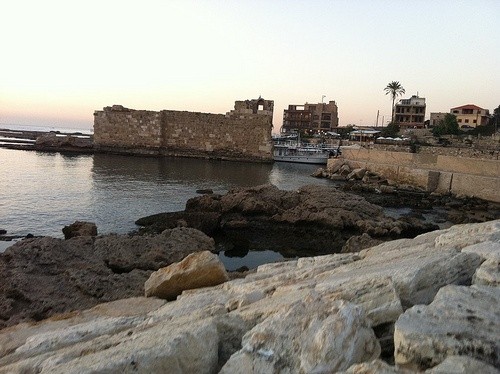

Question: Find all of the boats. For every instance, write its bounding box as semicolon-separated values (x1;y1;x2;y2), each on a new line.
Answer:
272;133;339;164
